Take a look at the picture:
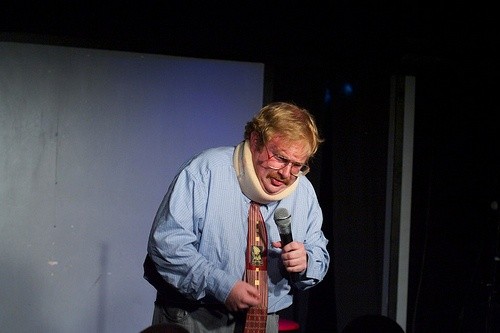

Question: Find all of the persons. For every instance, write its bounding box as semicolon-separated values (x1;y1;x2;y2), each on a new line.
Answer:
146;102;331;333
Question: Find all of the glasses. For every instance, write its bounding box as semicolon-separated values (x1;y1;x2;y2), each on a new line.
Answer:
259;133;310;177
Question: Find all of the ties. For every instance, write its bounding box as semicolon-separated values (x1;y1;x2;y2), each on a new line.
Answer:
245;202;268;333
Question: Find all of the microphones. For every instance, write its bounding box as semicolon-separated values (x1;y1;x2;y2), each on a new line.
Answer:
274;208;300;285
